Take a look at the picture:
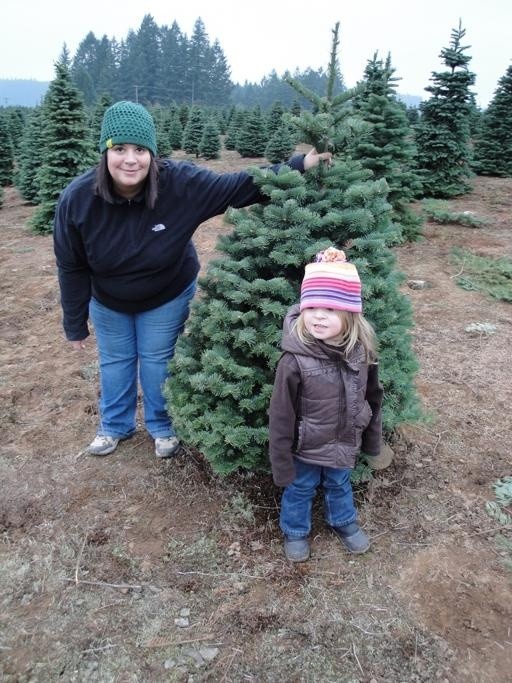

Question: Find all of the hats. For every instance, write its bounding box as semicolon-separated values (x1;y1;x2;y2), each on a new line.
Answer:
300;247;362;312
100;101;156;153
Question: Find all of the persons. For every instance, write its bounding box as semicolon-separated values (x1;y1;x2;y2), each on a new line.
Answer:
54;100;333;458
268;246;384;564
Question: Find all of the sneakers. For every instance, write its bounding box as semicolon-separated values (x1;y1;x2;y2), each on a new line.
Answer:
90;437;121;456
332;522;371;554
284;537;310;562
155;436;180;457
